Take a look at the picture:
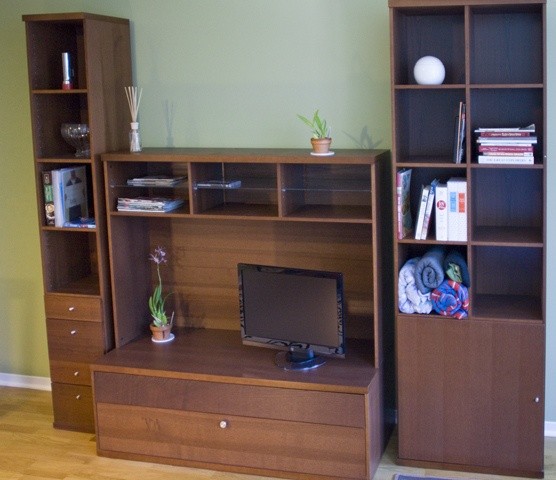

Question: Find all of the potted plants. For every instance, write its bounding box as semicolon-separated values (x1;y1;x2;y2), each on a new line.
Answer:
296;109;335;157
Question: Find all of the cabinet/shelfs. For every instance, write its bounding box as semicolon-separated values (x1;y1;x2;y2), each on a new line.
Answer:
90;327;367;480
394;314;546;479
388;0;547;322
101;147;392;394
41;294;103;433
22;13;134;295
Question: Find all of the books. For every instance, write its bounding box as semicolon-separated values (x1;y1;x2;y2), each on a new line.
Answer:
42;170;56;226
114;196;185;212
398;170;467;242
474;123;539;165
126;174;187;187
453;101;467;162
53;167;90;228
198;178;243;187
65;216;98;229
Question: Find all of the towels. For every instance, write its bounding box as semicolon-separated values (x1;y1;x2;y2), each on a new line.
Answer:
415;247;444;294
444;251;470;288
432;281;470;320
398;258;432;314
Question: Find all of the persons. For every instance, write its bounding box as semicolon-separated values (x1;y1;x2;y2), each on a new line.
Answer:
67;171;81;187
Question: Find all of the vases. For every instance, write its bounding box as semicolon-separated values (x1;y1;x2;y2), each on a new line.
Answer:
128;122;142;153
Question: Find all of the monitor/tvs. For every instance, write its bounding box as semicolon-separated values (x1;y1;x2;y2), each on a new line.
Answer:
236;263;345;372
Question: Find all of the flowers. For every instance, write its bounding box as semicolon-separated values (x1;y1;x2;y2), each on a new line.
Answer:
147;243;175;326
